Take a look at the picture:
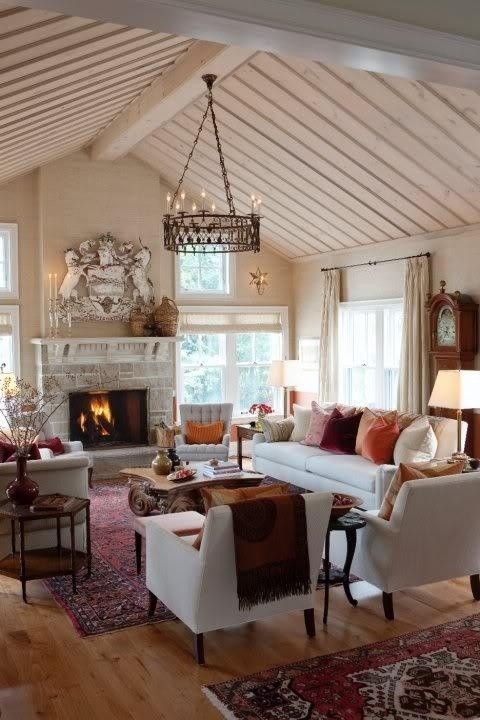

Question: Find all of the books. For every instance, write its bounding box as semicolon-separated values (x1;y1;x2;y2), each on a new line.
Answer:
204;460;241;480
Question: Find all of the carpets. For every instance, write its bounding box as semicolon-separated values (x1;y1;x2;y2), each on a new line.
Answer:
36;470;364;639
200;612;480;720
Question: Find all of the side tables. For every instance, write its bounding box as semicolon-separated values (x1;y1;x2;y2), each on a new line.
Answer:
0;493;91;603
236;425;263;470
323;510;367;624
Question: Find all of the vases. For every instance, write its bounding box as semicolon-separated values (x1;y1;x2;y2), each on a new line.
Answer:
256;414;267;428
7;457;40;509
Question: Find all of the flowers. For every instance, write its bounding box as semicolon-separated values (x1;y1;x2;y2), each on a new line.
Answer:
249;403;273;414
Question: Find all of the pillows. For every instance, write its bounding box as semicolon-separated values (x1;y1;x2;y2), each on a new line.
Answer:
259;414;295;443
289;403;337;442
38;437;65;456
319;408;363;455
364;415;399;465
185;420;223;444
191;482;290;550
393;417;438;466
377;460;466;520
0;441;42;462
355;407;398;455
300;401;356;446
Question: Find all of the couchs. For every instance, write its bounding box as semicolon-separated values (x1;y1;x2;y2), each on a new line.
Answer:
174;403;233;465
323;472;480;621
145;492;335;667
22;411;94;489
1;440;89;560
252;401;468;511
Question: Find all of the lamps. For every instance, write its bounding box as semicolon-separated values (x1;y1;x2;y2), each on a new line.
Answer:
427;370;479;471
161;75;264;256
268;360;302;417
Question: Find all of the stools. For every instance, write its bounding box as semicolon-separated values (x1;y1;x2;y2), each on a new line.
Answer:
134;511;206;575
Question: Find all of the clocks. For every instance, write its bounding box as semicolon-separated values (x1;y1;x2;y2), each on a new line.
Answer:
424;280;478;458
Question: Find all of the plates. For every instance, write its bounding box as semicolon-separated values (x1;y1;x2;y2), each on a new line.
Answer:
166;468;197;483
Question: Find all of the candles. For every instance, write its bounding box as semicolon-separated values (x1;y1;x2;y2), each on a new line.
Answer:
49;274;51;298
55;273;57;297
